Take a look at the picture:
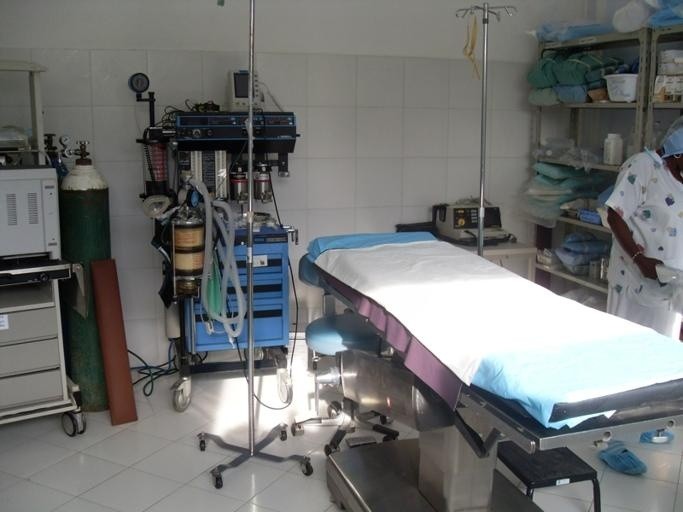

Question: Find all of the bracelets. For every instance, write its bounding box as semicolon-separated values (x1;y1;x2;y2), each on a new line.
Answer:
629;250;644;265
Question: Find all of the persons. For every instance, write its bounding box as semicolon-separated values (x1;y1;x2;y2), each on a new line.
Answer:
589;125;681;478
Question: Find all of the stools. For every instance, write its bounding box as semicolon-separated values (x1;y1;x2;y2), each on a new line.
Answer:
293;314;401;455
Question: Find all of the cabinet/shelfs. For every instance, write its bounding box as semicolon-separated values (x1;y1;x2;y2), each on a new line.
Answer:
648;24;683;156
531;28;648;303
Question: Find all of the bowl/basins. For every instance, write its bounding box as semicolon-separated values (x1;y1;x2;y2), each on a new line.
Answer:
602;73;639;104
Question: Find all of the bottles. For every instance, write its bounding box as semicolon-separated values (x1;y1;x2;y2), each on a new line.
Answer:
604;133;624;165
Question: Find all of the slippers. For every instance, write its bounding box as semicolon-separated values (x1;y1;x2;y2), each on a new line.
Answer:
640;430;674;442
599;441;647;475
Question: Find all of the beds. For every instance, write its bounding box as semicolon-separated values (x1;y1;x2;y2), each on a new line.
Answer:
297;229;683;510
